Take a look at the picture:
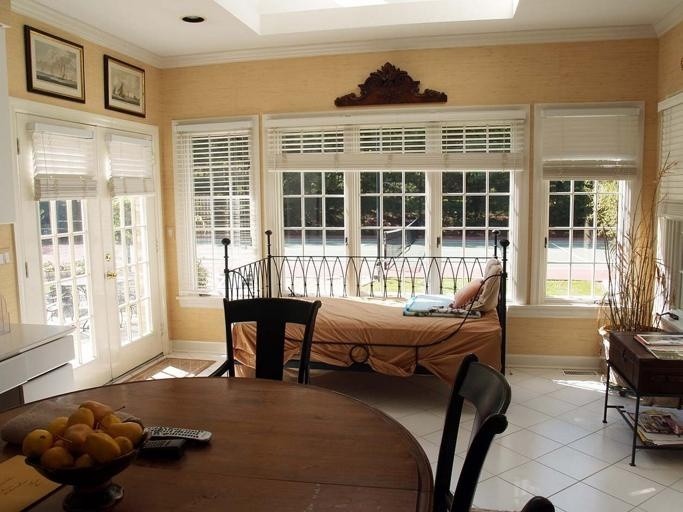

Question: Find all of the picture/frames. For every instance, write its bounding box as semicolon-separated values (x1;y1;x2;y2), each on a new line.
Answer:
23;24;85;103
103;54;145;118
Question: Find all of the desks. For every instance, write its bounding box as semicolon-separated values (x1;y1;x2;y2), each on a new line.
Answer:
0;377;433;512
602;331;683;467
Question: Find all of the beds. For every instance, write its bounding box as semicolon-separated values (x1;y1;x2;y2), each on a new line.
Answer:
221;230;509;411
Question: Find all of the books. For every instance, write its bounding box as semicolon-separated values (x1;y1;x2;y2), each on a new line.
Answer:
633;332;683;361
0;454;63;511
620;402;683;446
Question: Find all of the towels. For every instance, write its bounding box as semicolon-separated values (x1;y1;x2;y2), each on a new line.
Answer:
0;400;144;447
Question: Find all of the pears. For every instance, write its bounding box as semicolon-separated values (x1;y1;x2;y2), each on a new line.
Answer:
22;400;144;470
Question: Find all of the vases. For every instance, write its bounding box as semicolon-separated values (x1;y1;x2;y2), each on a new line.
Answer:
599;325;665;388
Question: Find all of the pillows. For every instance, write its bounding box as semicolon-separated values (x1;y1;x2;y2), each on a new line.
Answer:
452;276;484;309
452;258;503;312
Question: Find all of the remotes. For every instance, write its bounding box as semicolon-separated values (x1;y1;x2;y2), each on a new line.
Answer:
144;427;212;443
141;439;186;456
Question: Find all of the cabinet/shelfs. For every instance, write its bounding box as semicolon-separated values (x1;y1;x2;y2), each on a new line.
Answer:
0;324;76;403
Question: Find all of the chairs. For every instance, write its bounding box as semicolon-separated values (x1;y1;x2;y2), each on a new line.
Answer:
208;298;322;384
433;353;511;512
